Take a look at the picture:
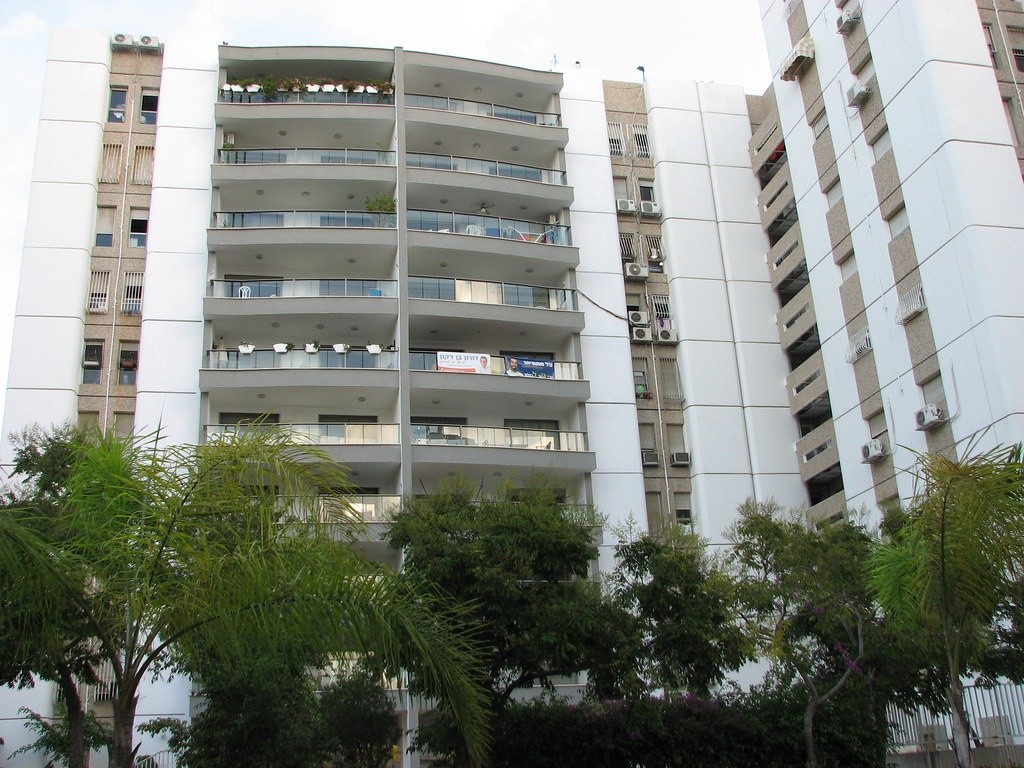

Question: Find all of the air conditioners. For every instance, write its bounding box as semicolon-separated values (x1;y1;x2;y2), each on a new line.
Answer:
628;311;647;325
639;200;661;216
671;451;690;465
915;404;938;431
630;327;652;344
623;263;649;283
138;35;159;50
656;328;677;345
227;134;234;145
846;80;867;105
546;214;556;228
110;33;133;47
642;452;660;465
837;10;853;32
616;199;637;214
861;439;883;463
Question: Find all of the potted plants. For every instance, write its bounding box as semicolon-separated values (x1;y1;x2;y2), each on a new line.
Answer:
120;359;133;368
333;344;352;354
221;73;396;102
304;339;320;353
272;342;295;352
365;339;384;354
238;341;255;353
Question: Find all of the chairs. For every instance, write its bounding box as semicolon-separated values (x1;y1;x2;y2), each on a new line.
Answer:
536;442;551;450
238;285;251;298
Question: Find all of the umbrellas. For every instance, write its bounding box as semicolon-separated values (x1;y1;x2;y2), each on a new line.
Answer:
215;337;229;368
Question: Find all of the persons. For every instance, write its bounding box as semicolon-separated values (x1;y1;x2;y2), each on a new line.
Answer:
505;357;524;376
475;356;491;373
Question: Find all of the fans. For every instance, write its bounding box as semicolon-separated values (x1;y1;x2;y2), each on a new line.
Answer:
471;201;495;215
